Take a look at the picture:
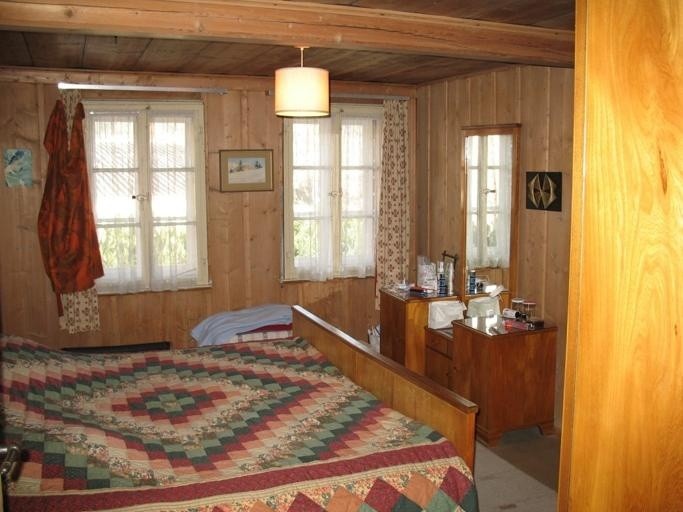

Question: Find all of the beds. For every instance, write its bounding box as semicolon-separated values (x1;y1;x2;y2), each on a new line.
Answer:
1;305;479;512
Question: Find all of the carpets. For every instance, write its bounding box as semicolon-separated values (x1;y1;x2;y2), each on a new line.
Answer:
473;440;558;512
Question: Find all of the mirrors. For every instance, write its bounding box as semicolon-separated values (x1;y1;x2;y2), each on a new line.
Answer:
461;123;521;313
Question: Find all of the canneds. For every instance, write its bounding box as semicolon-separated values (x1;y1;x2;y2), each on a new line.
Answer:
522;302;537;318
511;297;524;313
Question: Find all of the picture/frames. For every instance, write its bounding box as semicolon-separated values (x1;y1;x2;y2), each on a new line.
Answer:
219;149;275;192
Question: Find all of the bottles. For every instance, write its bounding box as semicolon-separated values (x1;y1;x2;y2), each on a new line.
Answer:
511;297;536;322
469;270;476;294
436;260;446;296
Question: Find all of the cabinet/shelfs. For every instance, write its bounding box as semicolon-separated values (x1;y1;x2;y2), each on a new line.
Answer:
379;286;457;376
424;316;558;449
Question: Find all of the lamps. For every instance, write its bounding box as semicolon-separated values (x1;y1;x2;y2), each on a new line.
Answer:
275;46;330;118
